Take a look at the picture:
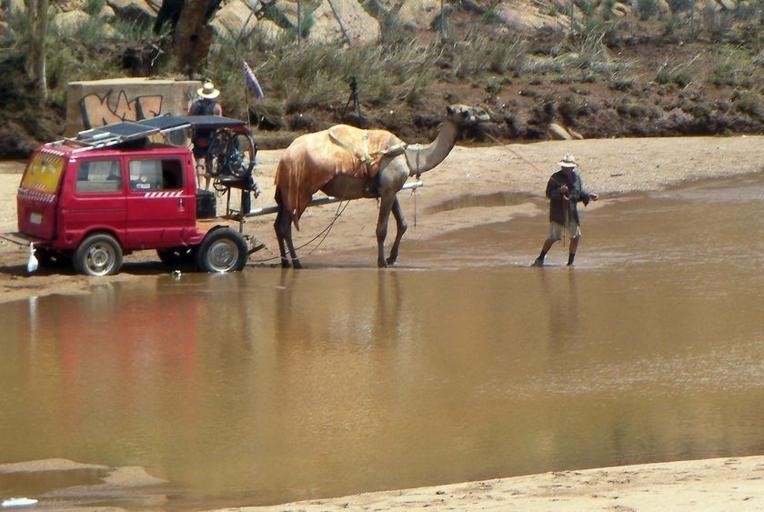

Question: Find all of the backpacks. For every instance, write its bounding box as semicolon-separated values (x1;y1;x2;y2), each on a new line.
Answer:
193;106;213;139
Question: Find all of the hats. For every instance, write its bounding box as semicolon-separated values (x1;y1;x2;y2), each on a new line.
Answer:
196;83;220;99
558;153;577;168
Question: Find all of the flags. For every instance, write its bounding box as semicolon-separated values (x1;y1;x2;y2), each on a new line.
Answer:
242;59;265;102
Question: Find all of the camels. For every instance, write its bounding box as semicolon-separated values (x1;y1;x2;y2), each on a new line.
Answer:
274;103;490;268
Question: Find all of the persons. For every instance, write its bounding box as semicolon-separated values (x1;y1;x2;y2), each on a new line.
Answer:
185;82;222;193
530;152;597;268
